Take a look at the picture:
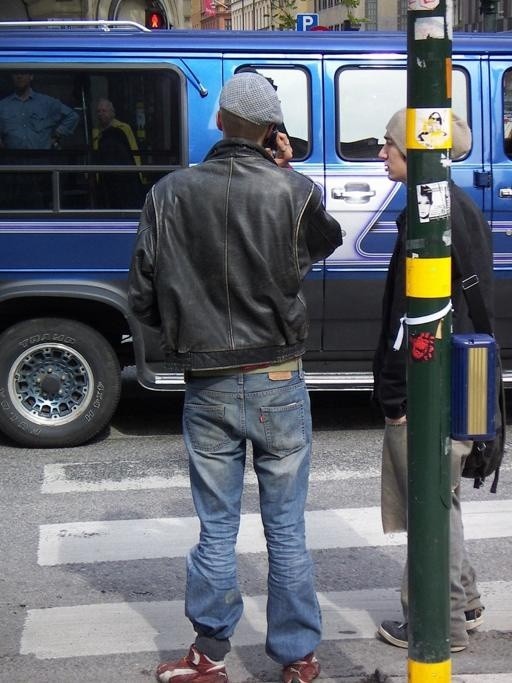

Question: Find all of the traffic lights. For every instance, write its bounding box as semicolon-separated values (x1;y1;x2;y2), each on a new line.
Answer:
147;9;165;30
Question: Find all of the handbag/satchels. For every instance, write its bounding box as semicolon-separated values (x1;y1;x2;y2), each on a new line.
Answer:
462;362;506;478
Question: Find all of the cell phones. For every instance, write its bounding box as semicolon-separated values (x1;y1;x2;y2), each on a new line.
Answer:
264;130;278;150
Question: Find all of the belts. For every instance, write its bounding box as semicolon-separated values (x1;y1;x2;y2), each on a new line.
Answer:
189;357;303;377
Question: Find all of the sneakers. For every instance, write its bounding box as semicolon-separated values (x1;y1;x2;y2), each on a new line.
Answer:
283;650;320;683
377;608;483;653
157;644;228;683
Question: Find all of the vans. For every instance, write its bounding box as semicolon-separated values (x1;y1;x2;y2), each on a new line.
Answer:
0;16;511;450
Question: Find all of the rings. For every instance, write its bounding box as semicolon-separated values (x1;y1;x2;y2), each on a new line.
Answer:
281;146;286;153
285;138;290;145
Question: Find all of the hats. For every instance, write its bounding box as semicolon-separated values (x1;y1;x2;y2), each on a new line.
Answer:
385;106;472;159
220;72;284;127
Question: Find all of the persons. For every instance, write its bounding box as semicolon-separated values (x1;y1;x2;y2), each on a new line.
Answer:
84;98;144;204
0;65;80;152
370;107;496;653
126;72;342;683
